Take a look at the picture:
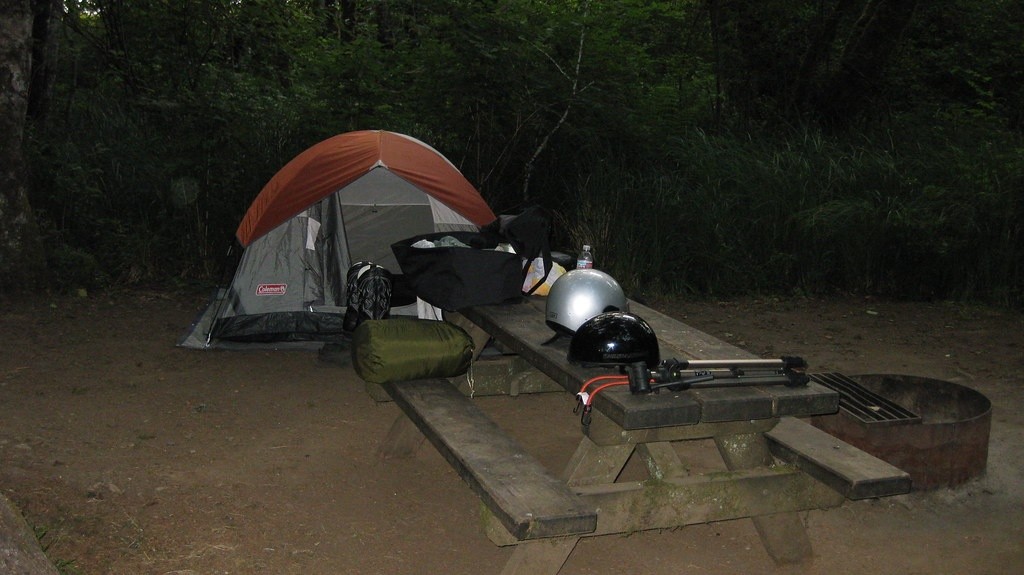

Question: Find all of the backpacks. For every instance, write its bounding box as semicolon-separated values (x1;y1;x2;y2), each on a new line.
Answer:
342;262;391;337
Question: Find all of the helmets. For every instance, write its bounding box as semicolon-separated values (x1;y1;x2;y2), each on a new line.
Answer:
568;312;661;368
545;269;629;336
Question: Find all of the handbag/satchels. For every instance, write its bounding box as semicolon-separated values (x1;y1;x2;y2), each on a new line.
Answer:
391;204;553;312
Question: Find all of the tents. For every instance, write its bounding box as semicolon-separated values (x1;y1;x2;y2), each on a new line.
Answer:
208;130;496;345
